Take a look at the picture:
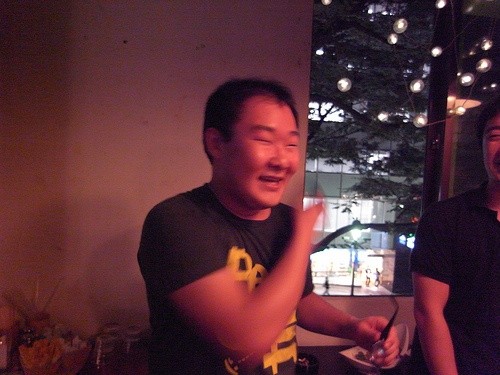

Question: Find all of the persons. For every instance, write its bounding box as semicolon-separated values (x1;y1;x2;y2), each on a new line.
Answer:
136;77;399;375
409;102;500;375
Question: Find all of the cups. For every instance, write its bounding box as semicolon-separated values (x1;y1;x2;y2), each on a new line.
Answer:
103;323;125;337
0;335;12;369
95;334;121;371
294;352;320;375
124;324;145;358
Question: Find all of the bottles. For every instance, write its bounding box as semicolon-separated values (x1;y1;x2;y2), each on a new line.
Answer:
22;328;34;344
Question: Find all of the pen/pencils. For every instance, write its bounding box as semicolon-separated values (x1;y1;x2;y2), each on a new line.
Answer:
377;306;399;341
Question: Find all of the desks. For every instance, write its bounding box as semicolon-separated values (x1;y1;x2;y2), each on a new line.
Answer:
0;340;429;375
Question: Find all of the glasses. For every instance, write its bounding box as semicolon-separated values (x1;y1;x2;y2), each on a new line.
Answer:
369;295;400;363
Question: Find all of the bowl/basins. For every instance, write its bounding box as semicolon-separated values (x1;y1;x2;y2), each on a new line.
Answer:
338;346;400;374
18;346;63;375
58;341;93;375
